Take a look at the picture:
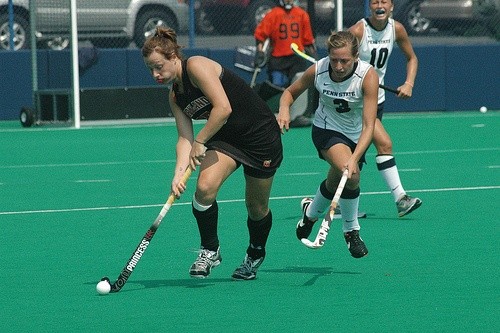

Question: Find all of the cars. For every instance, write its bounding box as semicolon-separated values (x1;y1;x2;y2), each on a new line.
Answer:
331;0;500;36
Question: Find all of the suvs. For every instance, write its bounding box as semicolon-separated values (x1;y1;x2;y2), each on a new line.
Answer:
0;0;280;51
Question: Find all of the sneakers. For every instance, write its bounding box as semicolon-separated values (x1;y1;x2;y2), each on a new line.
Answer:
396;194;423;217
296;198;318;240
231;253;265;279
344;230;368;258
189;245;222;278
333;205;366;219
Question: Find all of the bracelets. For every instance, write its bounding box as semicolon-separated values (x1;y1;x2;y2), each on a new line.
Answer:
406;81;414;88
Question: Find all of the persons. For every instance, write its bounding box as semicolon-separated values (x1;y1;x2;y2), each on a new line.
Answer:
253;0;422;258
141;27;283;280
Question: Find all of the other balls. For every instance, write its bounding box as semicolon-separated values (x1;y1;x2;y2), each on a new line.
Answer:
95;280;111;295
480;106;487;113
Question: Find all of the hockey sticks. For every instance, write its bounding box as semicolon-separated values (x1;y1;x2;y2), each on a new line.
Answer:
100;162;193;292
299;168;349;249
290;43;399;94
248;38;270;88
